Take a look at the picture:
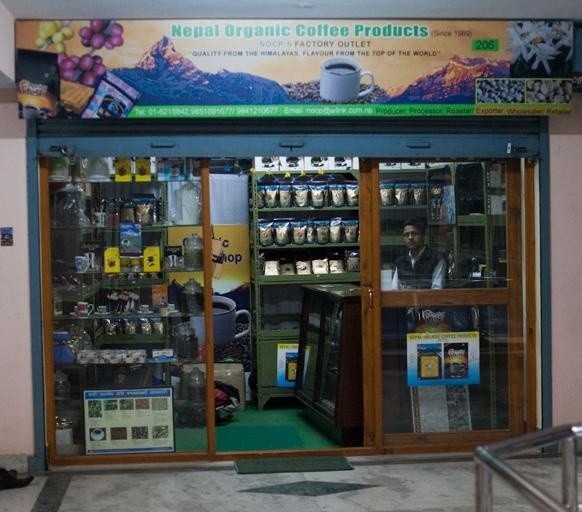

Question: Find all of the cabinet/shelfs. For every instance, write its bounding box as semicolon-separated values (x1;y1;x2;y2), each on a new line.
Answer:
247;161;509;443
38;145;210;456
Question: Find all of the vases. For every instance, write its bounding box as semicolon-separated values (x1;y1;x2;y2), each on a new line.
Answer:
58;79;96;114
511;50;566;78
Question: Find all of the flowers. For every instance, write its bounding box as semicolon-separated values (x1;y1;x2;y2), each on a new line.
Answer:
34;18;123;85
507;19;574;76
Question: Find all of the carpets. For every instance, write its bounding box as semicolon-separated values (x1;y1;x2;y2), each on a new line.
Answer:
233;452;354;475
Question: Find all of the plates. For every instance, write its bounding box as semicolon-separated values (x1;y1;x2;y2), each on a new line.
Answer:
94;312;111;317
136;310;154;315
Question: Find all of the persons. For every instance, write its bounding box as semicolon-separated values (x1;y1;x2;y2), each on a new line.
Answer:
390;215;448;332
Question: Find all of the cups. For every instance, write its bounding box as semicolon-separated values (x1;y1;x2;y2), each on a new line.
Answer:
76;300;95;319
93;211;106;228
74;255;91;273
158;307;170;317
97;305;107;314
167;303;175;311
181;235;204;270
212;294;252;349
139;304;150;312
168;309;180;314
